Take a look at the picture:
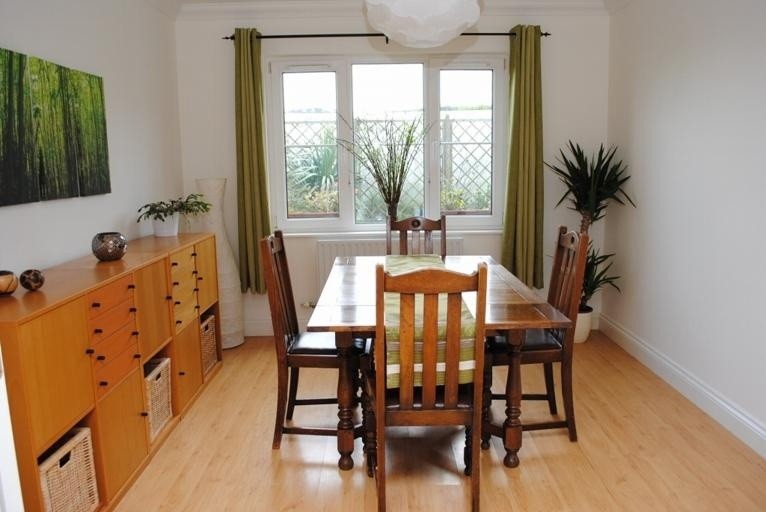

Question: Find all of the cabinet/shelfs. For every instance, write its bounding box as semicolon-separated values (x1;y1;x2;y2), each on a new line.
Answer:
0;297;88;458
128;252;173;373
169;243;204;337
154;318;204;417
75;368;150;507
85;273;149;409
193;236;218;315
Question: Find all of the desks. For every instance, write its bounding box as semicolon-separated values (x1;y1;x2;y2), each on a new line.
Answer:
302;251;572;471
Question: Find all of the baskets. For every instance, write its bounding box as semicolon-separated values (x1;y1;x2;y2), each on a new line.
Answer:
201;314;217;376
144;358;173;444
39;427;99;512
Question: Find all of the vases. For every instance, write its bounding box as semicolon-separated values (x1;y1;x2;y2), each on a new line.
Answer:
190;177;246;350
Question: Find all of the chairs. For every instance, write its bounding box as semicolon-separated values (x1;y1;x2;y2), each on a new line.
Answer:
258;230;367;451
364;262;489;511
381;215;451;256
481;224;590;449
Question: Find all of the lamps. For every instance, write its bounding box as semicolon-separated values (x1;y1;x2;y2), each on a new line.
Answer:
356;0;482;49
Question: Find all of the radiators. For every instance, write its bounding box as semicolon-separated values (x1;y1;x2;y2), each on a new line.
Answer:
301;235;465;307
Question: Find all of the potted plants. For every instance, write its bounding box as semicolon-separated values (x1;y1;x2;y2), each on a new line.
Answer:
137;193;206;236
541;137;635;343
